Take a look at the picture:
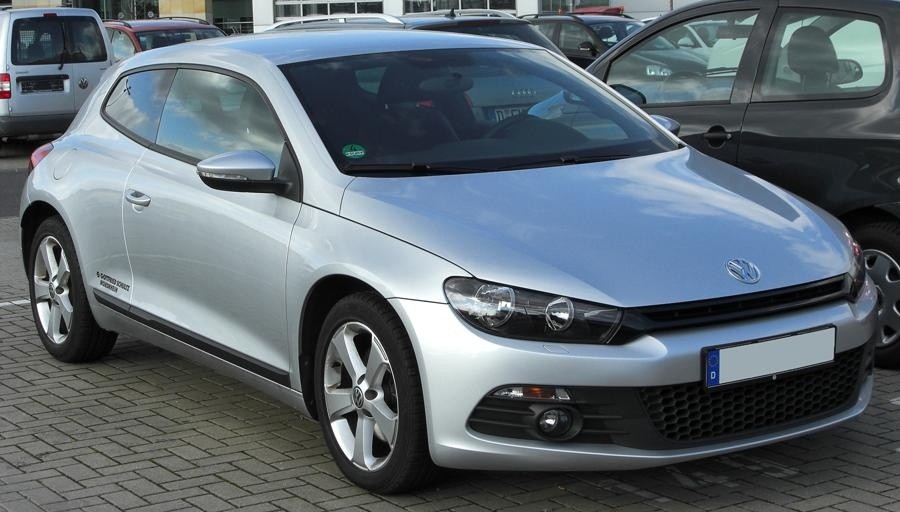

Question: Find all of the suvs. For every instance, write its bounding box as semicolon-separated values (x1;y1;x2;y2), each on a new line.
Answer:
516;12;708;83
100;16;229;64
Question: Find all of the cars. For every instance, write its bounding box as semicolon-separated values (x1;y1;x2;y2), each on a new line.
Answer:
261;9;617;126
18;23;879;496
600;15;754;64
528;1;900;371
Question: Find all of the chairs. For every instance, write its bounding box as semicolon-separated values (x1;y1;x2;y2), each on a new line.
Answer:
28;44;44;60
378;60;479;150
242;68;376;150
788;26;841;94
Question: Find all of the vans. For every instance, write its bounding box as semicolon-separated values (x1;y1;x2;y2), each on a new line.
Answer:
710;12;886;89
0;7;116;159
